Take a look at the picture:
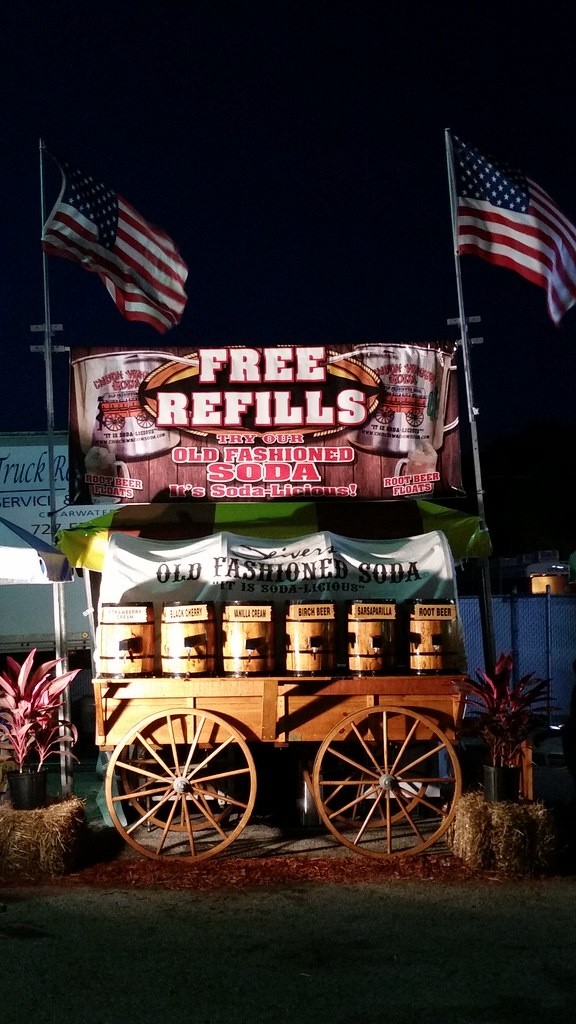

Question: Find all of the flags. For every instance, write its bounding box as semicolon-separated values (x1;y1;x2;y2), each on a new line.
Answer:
447;127;576;326
41;146;189;336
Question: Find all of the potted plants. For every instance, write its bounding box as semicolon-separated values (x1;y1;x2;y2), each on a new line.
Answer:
450;651;568;805
1;645;78;807
